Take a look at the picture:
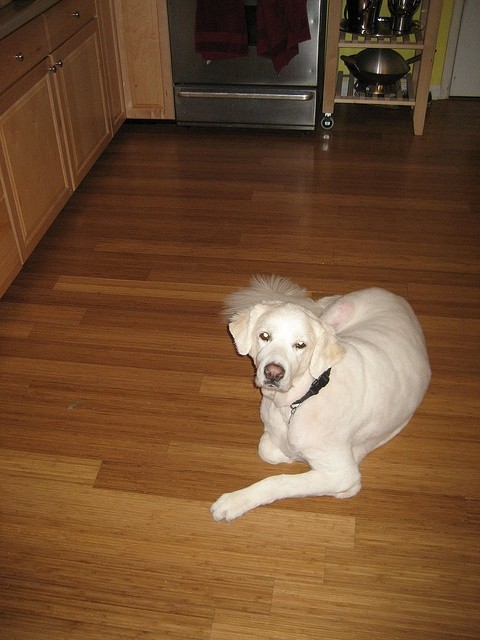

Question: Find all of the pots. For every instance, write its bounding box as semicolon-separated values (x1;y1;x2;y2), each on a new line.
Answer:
340;48;410;75
345;53;422;84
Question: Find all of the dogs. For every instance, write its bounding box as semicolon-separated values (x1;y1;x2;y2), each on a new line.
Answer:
208;272;432;524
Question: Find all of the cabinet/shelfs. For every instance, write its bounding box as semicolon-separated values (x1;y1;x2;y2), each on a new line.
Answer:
321;0;443;137
0;0;126;266
111;0;183;143
0;136;26;299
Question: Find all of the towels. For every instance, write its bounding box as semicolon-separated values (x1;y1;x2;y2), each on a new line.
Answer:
253;0;312;75
195;0;250;61
